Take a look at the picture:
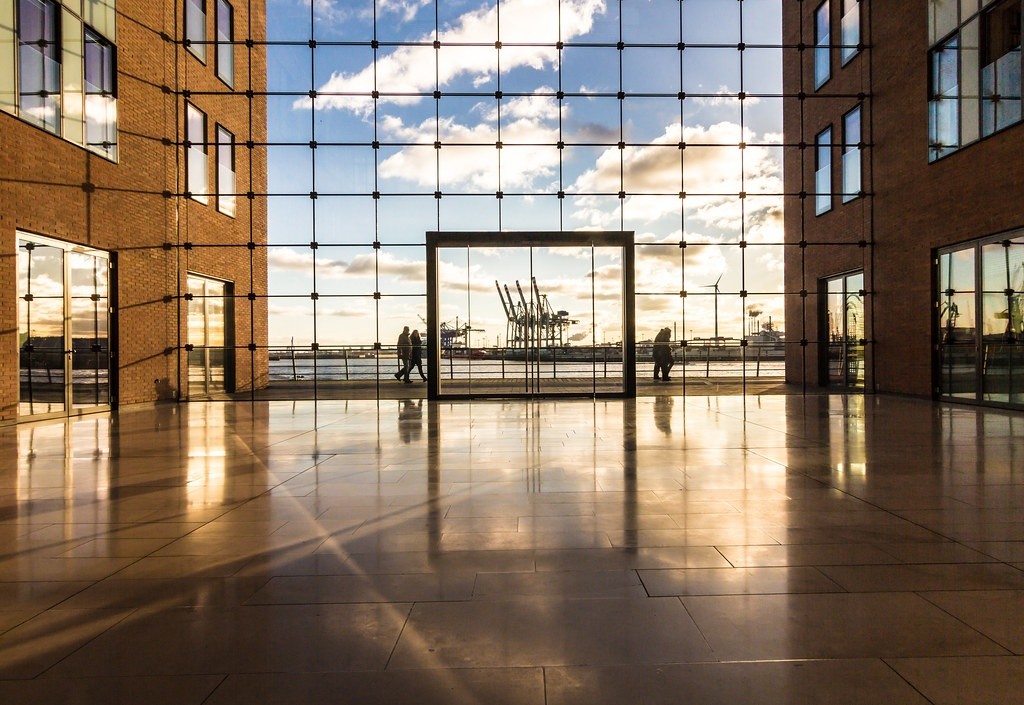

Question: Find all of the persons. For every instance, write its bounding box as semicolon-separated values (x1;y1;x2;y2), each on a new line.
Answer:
404;330;427;382
653;328;674;380
394;326;413;383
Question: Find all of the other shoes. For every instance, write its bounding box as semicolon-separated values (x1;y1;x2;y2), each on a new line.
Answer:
403;379;410;381
394;373;401;381
422;378;427;382
404;381;413;384
653;376;673;382
